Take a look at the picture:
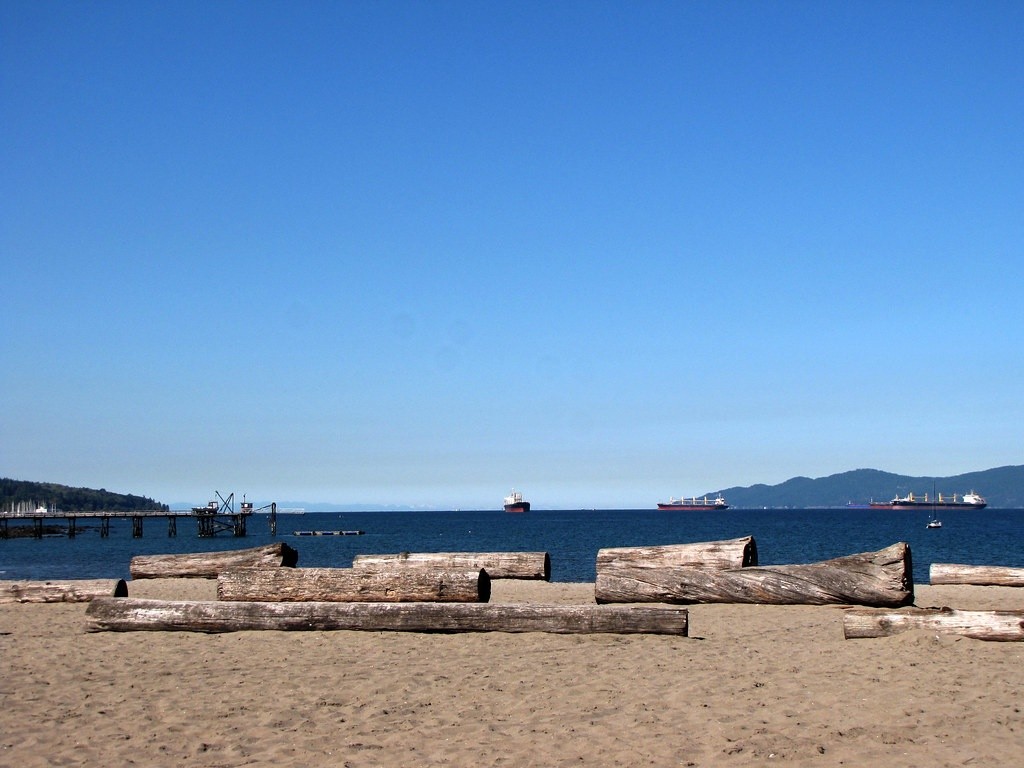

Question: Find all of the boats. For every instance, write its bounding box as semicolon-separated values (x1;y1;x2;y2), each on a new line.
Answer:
657;494;728;511
502;491;531;513
869;491;988;511
240;503;308;515
924;517;942;532
